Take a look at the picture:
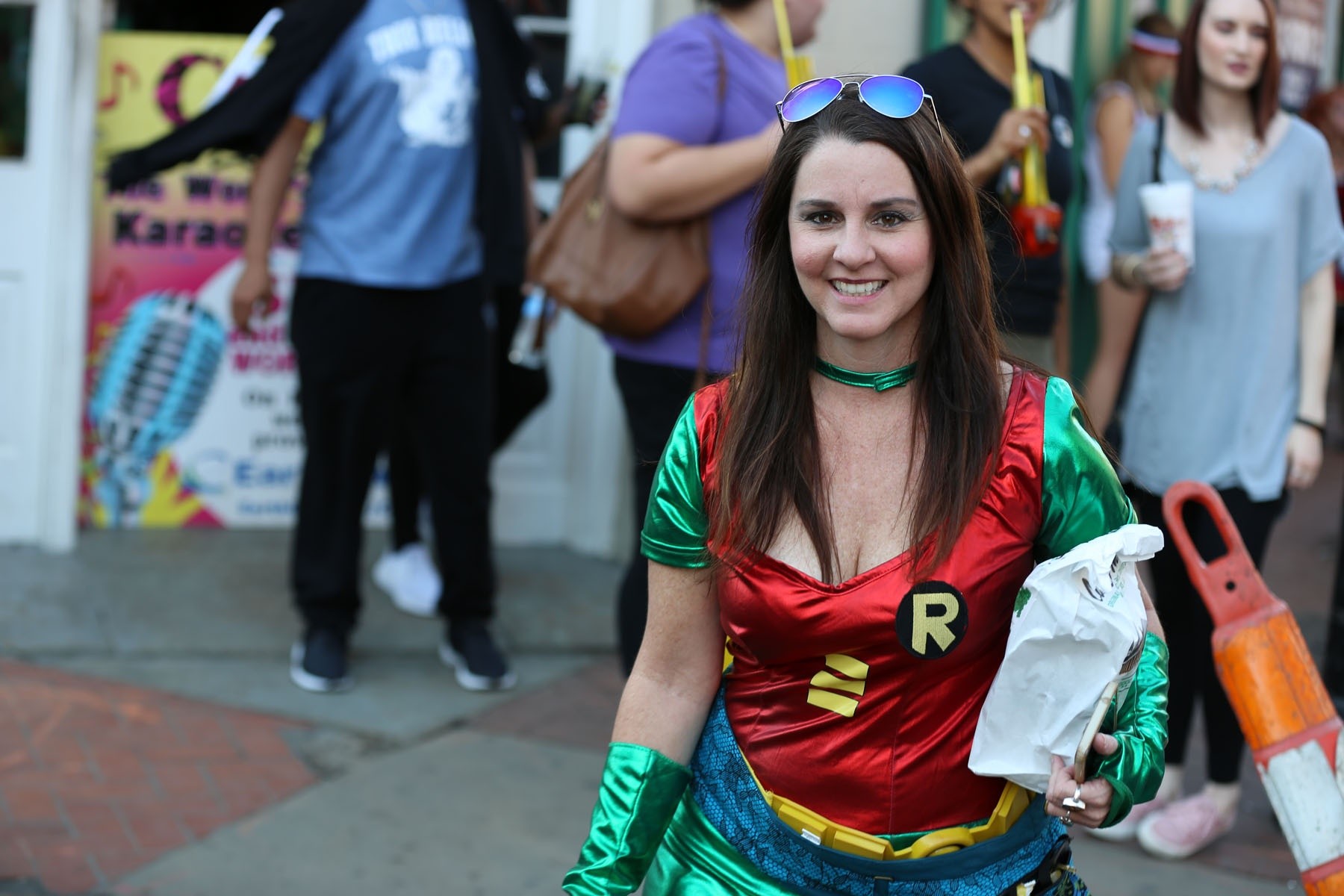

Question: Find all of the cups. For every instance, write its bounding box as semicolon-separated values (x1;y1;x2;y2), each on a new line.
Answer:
1139;180;1194;268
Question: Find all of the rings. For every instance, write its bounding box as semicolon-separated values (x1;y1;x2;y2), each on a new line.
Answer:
1020;125;1032;140
1062;781;1086;812
1059;809;1073;826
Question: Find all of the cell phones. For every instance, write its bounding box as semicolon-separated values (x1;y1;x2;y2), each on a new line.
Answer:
1074;682;1120;784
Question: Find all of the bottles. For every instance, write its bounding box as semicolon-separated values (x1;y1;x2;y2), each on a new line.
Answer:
509;284;556;367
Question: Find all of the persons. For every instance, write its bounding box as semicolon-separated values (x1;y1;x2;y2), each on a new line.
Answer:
227;0;606;692
609;0;825;688
1086;1;1344;861
894;0;1075;379
565;70;1167;896
1301;86;1344;224
1082;12;1182;444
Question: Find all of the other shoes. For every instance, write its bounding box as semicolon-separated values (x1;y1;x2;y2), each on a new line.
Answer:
1085;782;1235;859
290;631;353;691
440;629;516;689
371;544;442;615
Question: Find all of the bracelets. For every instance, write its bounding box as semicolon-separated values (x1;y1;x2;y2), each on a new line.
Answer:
1292;416;1329;437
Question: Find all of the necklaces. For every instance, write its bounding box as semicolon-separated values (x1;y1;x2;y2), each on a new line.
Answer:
810;356;922;391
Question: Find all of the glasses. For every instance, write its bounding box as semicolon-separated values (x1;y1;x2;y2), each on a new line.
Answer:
773;72;944;144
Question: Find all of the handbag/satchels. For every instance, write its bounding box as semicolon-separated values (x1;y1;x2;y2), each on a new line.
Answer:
524;130;708;341
